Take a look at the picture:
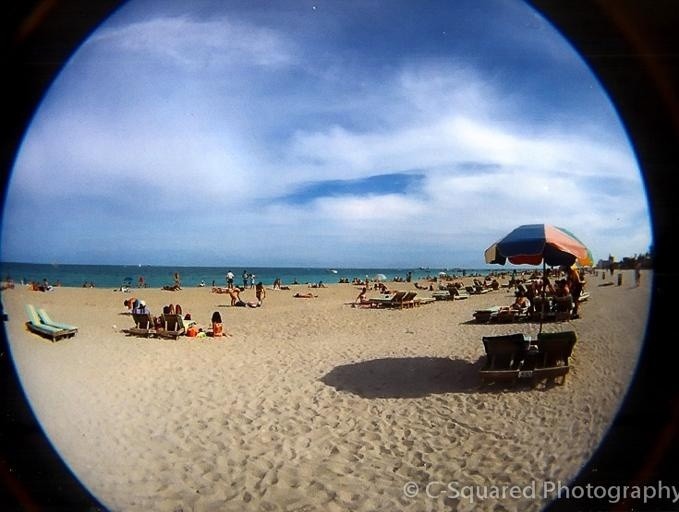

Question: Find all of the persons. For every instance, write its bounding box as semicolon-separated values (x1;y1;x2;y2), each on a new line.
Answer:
139;276;144;285
133;300;151;316
124;297;139;314
510;264;589;316
163;284;182;291
255;282;266;305
162;303;183;317
293;292;318;298
355;287;370;304
229;287;244;306
174;272;180;284
352;277;387;293
226;270;235;288
632;260;643;287
318;280;328;289
391;268;509;296
273;278;280;288
210;311;224;336
249;273;256;288
241;269;249;289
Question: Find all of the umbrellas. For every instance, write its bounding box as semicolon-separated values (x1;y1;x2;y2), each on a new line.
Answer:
483;223;594;333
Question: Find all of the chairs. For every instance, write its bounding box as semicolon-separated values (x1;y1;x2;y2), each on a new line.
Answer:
517;332;576;388
24;303;64;342
37;308;79;338
123;309;162;339
368;275;573;324
160;313;195;341
478;333;533;388
